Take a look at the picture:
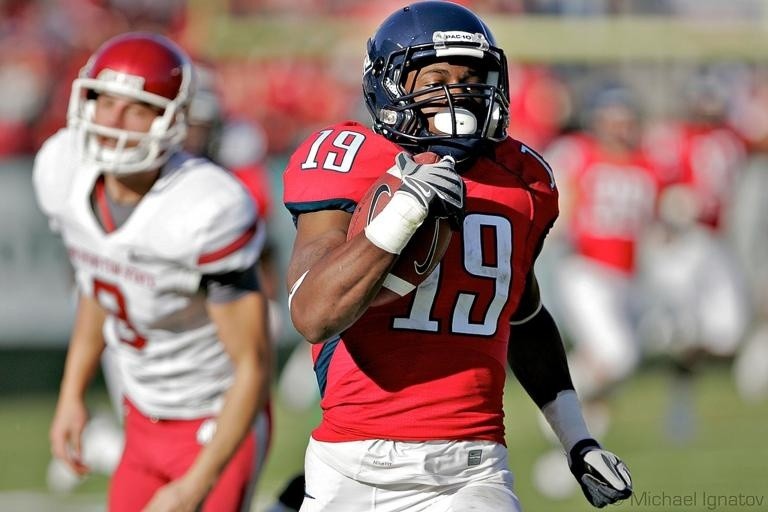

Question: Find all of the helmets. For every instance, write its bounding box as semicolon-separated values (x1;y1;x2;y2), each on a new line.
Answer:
66;31;193;175
362;2;509;153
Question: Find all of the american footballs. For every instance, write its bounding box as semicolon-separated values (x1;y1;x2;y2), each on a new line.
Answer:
345;152;452;307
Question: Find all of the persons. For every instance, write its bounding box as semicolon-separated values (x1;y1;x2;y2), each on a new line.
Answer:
282;1;634;511
529;80;699;498
31;26;274;510
0;1;768;418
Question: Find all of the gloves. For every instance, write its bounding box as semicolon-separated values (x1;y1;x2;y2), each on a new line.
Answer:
394;151;468;217
567;439;633;508
